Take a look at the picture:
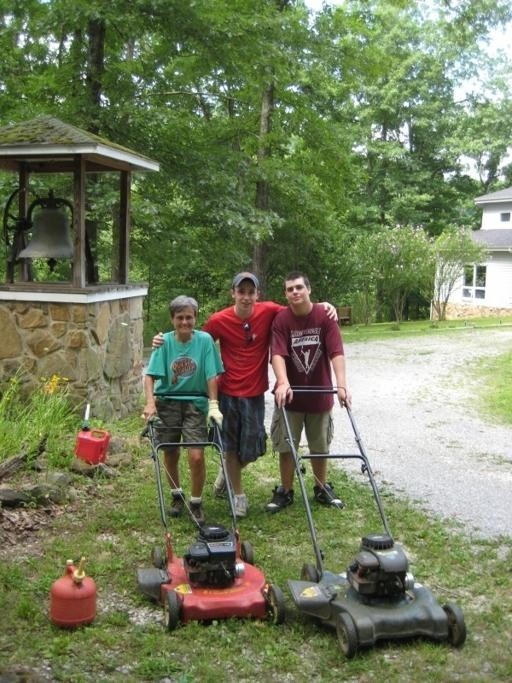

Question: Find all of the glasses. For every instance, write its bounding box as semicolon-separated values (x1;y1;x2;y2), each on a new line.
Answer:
240;319;253;346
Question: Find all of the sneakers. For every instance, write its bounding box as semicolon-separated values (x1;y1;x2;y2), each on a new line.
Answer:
312;483;345;511
213;468;226;499
228;493;249;518
168;492;185;518
188;500;206;526
265;484;295;513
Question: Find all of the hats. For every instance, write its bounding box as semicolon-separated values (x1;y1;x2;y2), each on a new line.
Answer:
232;270;260;290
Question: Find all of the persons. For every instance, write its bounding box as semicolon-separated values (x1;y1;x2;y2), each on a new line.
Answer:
262;270;353;514
150;270;340;519
142;294;227;526
